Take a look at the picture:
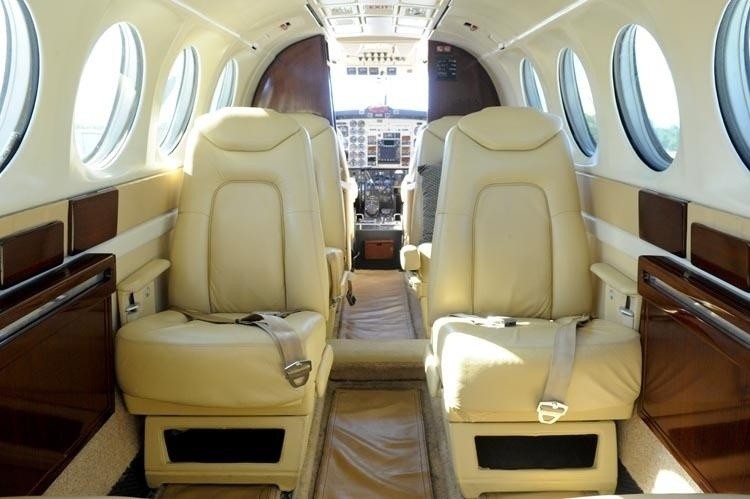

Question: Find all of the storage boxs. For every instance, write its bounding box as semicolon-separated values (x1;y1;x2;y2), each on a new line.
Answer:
365;240;395;260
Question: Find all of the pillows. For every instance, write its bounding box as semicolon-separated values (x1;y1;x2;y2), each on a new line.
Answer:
416;158;445;242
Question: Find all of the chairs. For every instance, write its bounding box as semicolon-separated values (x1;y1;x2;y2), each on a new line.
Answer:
279;108;350;333
112;106;336;494
333;125;359;245
421;104;646;495
400;112;465;302
398;125;425;242
